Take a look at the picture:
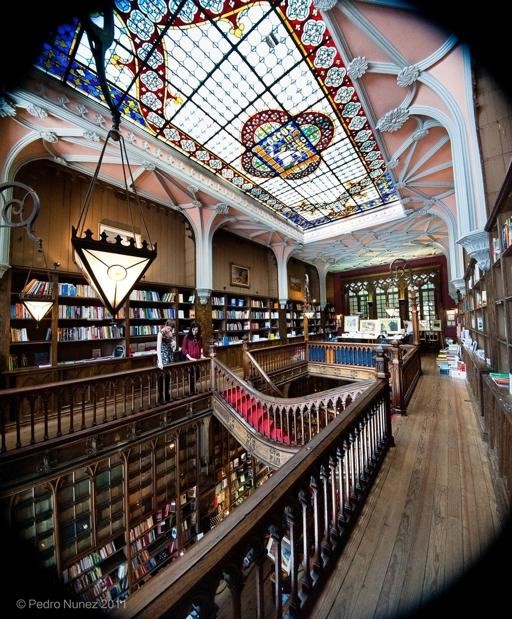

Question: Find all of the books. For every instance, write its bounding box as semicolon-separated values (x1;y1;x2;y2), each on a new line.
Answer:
339;329;404;340
436;288;493;378
9;279;196;369
211;297;334;346
489;373;509;389
60;449;275;619
491;216;512;264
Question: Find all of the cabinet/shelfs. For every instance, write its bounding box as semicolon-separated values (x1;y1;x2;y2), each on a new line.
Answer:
0;413;276;612
9;264;338;431
419;330;445;356
454;158;511;551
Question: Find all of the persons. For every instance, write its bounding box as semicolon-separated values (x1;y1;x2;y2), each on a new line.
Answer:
182;322;208;397
156;319;177;403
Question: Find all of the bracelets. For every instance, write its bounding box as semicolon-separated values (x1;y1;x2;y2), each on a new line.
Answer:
201;353;203;355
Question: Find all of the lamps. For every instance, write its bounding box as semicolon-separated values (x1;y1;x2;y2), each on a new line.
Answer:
71;128;157;326
